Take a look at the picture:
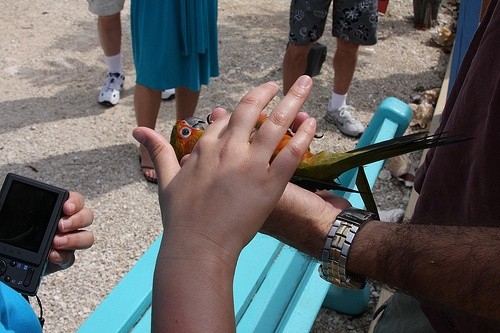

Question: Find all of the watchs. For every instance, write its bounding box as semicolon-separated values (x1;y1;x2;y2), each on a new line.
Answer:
317;206;377;291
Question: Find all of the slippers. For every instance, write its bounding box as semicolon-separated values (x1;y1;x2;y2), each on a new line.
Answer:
139;156;157;183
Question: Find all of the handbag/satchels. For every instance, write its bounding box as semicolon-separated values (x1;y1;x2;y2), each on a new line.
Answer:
286;40;327;77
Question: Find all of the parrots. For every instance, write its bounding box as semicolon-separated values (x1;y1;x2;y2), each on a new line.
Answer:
170;112;479;193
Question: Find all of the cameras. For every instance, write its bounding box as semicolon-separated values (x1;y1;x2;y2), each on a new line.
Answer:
0;173;69;296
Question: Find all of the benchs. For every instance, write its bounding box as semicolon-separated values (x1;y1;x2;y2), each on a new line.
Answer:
74;97;412;333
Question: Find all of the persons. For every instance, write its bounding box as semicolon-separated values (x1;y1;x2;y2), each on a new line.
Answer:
0;0;500;333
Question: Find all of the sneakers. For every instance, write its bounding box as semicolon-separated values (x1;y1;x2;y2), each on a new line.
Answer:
161;88;175;100
325;100;365;136
98;73;125;106
